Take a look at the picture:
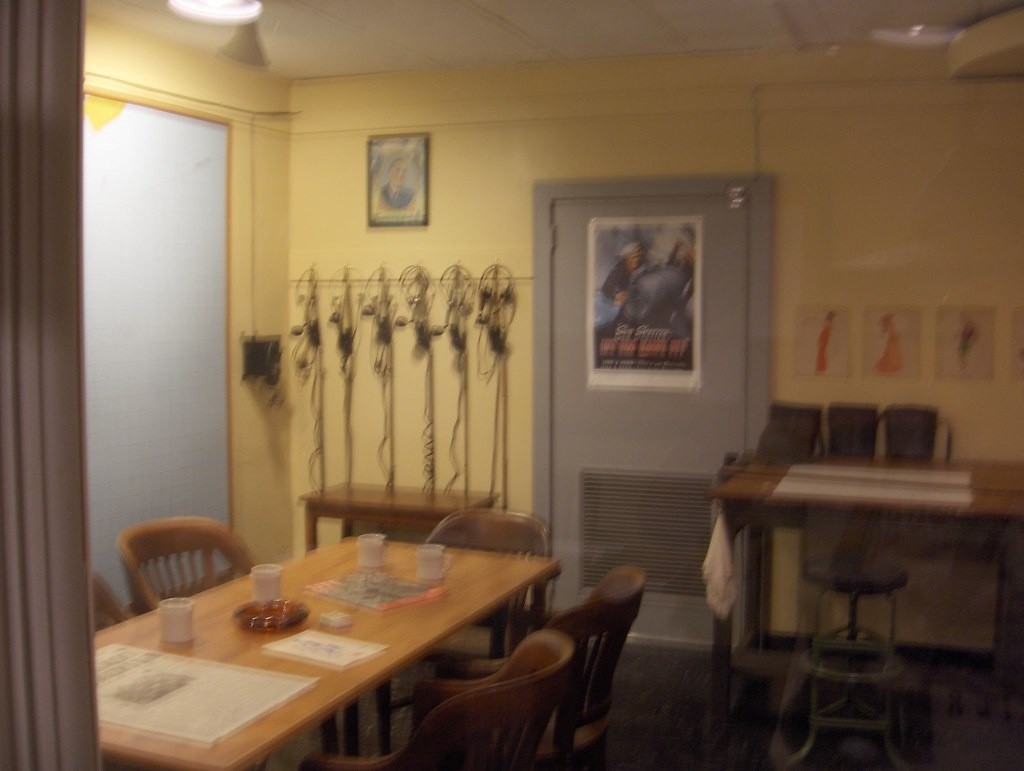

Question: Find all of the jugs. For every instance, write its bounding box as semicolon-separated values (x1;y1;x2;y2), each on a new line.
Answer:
830;402;885;457
885;404;954;464
767;397;824;460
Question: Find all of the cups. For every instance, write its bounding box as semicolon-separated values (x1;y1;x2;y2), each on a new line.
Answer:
250;564;282;603
155;597;193;643
416;544;454;580
356;534;386;569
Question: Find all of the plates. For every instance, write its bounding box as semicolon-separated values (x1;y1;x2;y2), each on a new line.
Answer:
234;600;309;628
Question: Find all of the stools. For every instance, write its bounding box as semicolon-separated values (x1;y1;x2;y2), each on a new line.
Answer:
784;554;916;769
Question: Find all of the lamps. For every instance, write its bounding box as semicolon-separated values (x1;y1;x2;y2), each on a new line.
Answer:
170;0;262;30
867;23;967;50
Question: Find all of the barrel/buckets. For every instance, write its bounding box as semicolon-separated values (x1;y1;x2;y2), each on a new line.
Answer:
622;268;685;320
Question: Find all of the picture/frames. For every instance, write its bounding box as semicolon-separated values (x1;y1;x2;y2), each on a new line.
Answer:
365;132;430;228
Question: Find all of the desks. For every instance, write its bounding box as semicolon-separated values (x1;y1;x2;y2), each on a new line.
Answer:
696;452;1023;765
94;532;568;770
297;480;499;560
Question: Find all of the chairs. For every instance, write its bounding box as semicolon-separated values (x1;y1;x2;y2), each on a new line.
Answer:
293;629;575;771
90;562;141;634
433;558;645;770
113;516;266;616
406;511;552;695
